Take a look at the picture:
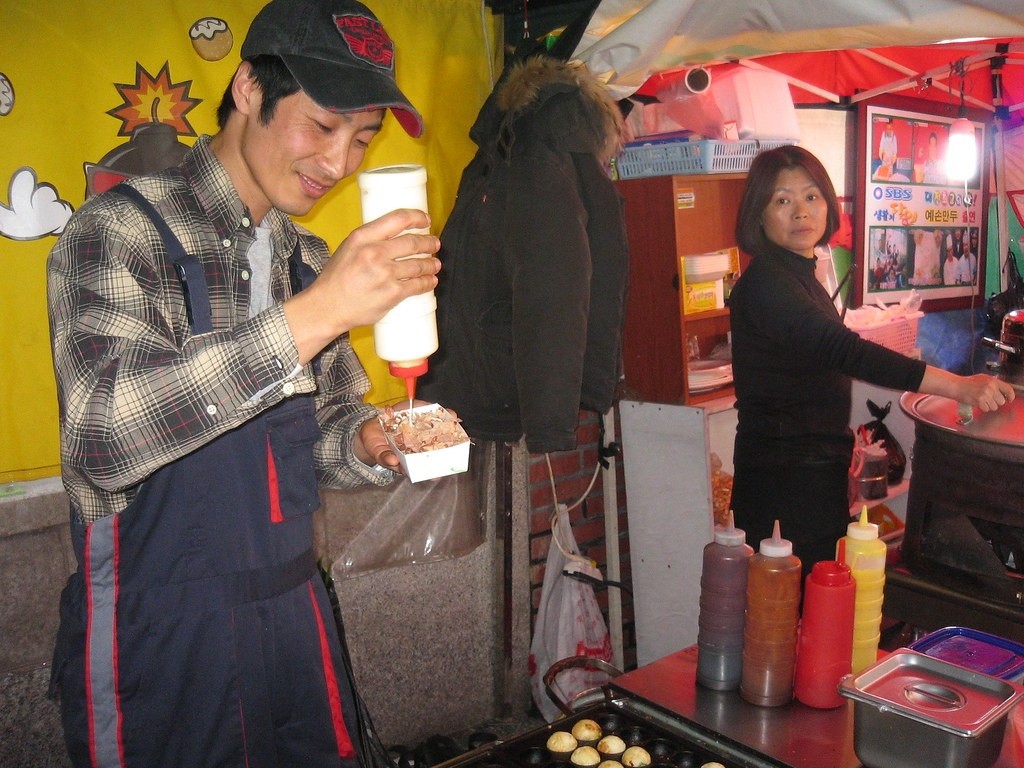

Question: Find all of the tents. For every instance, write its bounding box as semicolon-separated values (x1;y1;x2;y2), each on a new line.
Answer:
546;1;1024;360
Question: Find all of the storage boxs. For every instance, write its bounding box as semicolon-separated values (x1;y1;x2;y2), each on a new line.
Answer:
836;625;1024;768
378;402;471;484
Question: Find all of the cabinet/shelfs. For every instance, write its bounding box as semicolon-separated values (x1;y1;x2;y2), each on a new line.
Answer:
622;348;921;668
612;172;750;405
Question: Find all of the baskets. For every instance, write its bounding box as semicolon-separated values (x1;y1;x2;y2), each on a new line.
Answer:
850;311;924;353
617;138;799;180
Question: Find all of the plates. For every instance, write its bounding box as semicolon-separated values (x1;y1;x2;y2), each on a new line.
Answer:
685;254;730;282
687;359;734;393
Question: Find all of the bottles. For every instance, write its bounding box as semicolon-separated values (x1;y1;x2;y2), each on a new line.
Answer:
691;510;751;690
749;521;802;708
796;541;855;709
834;504;888;671
357;166;439;402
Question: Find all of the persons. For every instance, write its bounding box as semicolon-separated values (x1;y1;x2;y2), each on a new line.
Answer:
44;1;445;768
727;144;1015;616
869;117;980;286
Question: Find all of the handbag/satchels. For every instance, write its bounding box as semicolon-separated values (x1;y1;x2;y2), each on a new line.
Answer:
528;503;618;724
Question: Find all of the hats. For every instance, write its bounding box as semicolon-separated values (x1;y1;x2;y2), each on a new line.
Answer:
239;0;425;139
